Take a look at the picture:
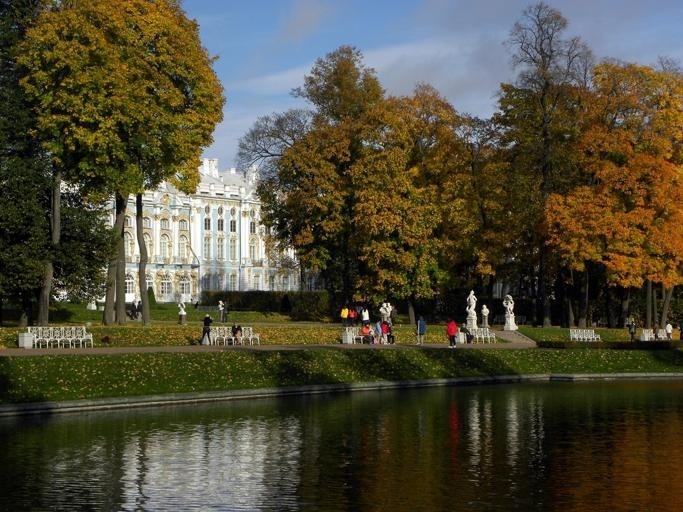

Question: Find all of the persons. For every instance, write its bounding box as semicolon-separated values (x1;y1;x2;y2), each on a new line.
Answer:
200;314;213;345
341;303;396;345
223;300;228;322
218;301;225;322
666;320;673;340
446;320;459;348
131;301;142;320
653;320;659;339
460;323;474;344
232;322;242;336
416;316;426;346
629;321;636;342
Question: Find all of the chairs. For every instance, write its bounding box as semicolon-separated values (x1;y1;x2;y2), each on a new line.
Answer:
346;326;367;344
642;328;672;340
569;329;600;341
205;325;261;347
27;326;94;349
458;326;497;345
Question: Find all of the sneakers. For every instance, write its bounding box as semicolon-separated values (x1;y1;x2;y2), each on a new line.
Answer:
448;346;456;348
